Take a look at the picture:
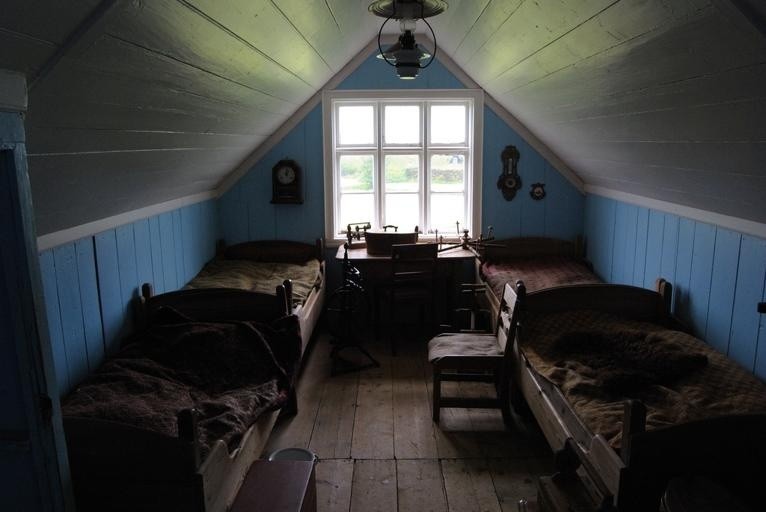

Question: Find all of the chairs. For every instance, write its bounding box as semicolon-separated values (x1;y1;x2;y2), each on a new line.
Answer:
372;241;439;358
426;279;527;430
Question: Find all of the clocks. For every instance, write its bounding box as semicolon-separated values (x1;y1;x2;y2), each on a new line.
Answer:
269;160;304;205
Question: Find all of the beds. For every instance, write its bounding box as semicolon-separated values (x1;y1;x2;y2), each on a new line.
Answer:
62;282;300;512
511;280;766;512
142;237;330;383
479;232;666;354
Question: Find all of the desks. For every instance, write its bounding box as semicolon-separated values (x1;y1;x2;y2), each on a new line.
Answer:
334;242;476;309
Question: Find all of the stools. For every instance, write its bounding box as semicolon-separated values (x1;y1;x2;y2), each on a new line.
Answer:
230;458;317;512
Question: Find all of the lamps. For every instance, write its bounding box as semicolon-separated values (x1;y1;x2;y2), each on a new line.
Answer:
367;0;449;80
